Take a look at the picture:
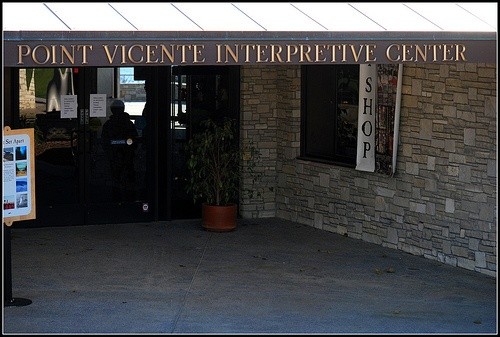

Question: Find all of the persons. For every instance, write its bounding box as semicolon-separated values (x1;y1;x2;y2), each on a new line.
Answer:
100;100;140;207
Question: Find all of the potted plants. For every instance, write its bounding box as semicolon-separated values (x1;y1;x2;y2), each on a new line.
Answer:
183;115;275;235
